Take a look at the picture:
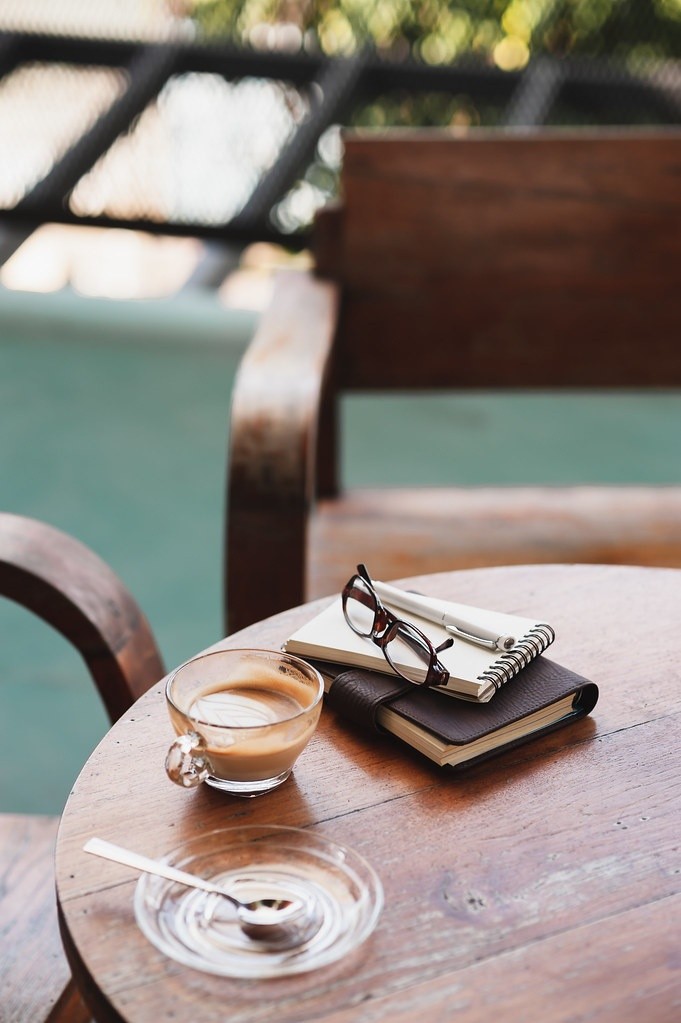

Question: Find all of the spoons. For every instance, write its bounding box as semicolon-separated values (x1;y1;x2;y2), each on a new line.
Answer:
83;837;306;927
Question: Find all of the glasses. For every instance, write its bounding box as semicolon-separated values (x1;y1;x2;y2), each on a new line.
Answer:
341;564;454;689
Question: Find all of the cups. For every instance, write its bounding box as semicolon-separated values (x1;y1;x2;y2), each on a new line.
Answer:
164;647;324;797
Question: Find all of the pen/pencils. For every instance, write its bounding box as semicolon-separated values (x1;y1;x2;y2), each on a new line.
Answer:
362;577;516;654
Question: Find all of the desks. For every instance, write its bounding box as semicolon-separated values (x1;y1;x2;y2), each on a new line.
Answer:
55;562;681;1023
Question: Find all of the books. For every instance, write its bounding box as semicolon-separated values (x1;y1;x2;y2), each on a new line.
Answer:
280;576;555;704
279;590;599;774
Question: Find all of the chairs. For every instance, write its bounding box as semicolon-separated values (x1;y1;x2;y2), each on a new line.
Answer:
0;513;167;1022
224;125;681;637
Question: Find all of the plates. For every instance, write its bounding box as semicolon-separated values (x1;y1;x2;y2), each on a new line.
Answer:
133;824;385;979
